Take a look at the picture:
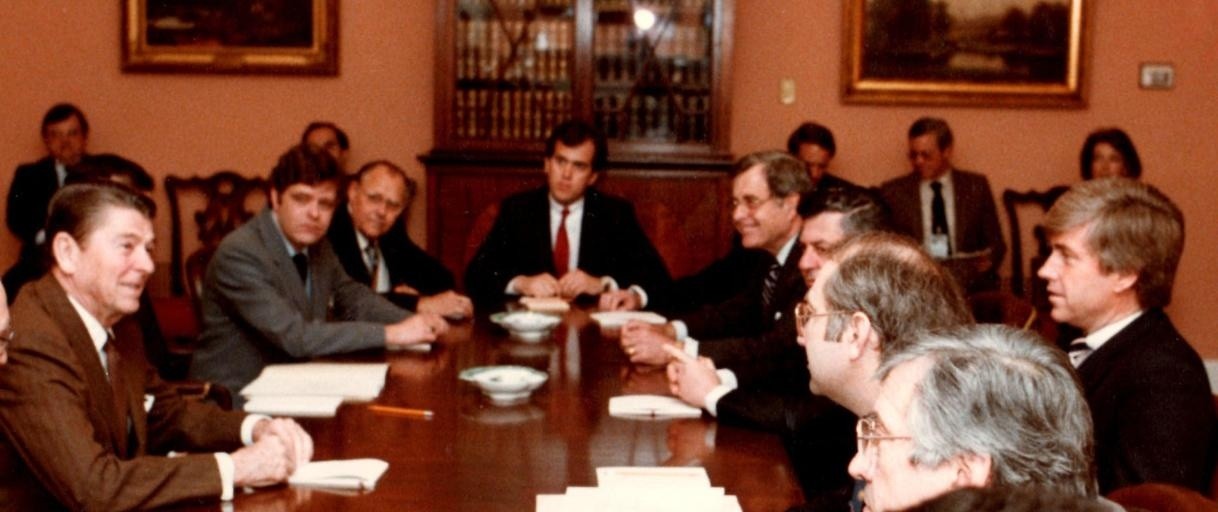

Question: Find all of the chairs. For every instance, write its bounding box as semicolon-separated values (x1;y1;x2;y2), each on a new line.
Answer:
1000;185;1076;313
151;171;275;357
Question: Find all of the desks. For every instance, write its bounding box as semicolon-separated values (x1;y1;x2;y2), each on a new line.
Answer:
210;304;808;510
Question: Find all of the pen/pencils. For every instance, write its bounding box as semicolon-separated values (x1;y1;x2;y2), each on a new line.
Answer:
367;405;435;416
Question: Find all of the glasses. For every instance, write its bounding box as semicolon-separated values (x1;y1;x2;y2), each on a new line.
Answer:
795;302;851;331
731;195;774;208
857;419;912;453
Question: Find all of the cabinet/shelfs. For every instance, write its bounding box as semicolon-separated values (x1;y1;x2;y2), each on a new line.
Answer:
413;1;738;298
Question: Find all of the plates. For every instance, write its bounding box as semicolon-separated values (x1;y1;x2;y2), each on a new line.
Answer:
453;364;549;404
490;309;562;344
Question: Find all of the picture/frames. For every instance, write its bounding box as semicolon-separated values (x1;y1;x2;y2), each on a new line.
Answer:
836;0;1099;110
117;0;342;79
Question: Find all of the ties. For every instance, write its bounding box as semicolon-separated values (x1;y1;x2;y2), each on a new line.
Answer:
926;180;952;258
362;243;379;290
554;207;571;279
101;334;127;456
758;261;779;304
290;251;309;288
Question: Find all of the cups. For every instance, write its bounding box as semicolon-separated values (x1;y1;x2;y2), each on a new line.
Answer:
925;233;949;258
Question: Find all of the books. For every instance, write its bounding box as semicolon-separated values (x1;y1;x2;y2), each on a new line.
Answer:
456;0;713;139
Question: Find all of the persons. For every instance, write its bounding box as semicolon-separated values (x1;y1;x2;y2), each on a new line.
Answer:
848;322;1098;512
459;117;673;309
1037;176;1218;501
661;185;859;465
1078;127;1143;179
786;121;837;189
190;148;454;405
0;181;314;512
598;114;1214;511
0;103;93;308
793;231;975;416
878;116;1009;290
594;150;814;362
299;120;356;240
326;161;474;318
